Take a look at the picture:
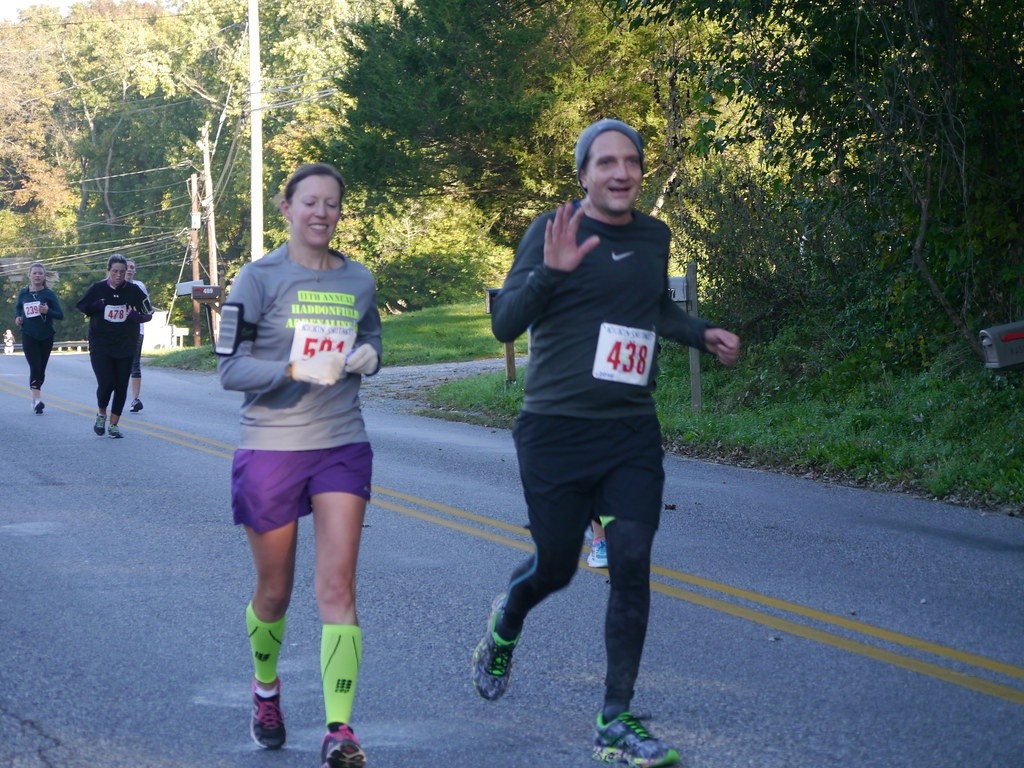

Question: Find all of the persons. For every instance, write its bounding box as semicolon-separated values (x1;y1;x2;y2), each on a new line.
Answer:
15;263;63;414
217;164;383;768
124;258;152;412
76;254;155;439
473;119;739;768
3;329;15;354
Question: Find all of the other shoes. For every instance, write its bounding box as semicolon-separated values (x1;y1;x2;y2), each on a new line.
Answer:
34;400;45;414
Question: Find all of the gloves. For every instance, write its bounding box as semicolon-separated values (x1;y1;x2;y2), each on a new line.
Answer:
290;352;344;385
345;344;379;375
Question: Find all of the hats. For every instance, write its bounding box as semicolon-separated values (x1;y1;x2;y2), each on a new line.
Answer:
575;120;644;194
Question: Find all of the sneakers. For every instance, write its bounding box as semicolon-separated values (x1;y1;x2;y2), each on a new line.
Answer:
250;676;286;749
94;413;107;436
108;424;123;438
586;538;608;568
130;398;143;412
321;723;367;768
470;591;523;702
591;709;681;768
